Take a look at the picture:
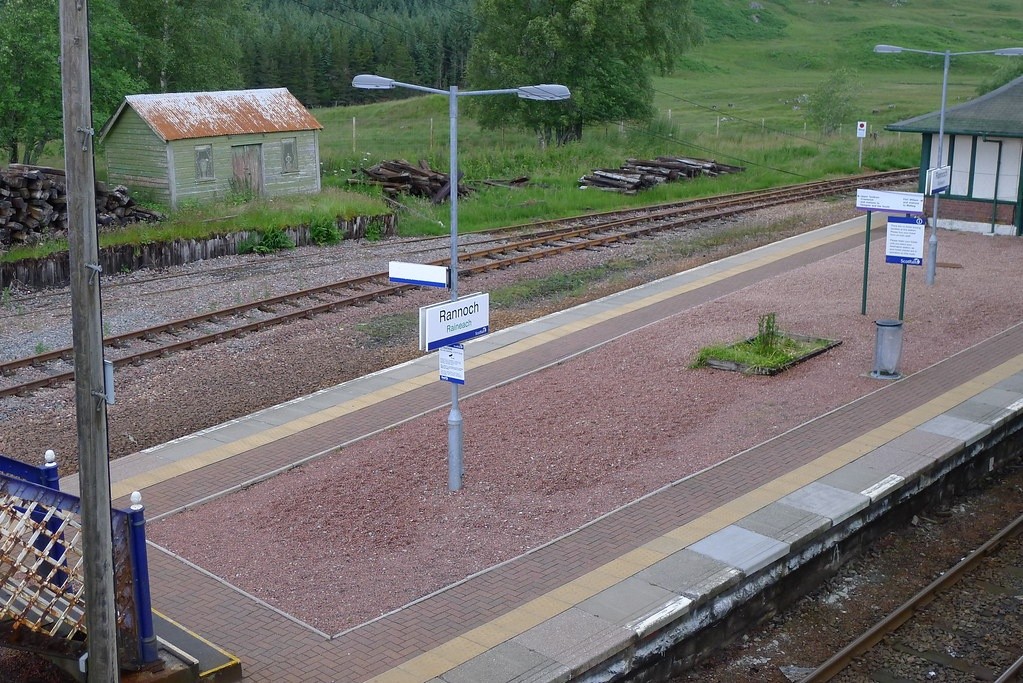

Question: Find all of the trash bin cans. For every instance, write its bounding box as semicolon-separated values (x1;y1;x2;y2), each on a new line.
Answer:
870;319;904;380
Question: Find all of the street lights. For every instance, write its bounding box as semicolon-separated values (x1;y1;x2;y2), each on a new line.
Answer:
351;73;571;490
873;44;1023;285
